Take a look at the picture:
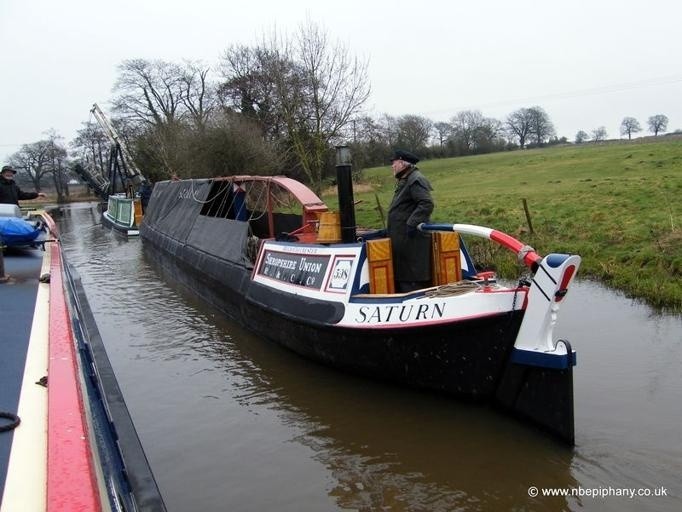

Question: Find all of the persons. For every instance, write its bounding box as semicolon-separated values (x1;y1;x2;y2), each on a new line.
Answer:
0;165;47;208
388;151;434;293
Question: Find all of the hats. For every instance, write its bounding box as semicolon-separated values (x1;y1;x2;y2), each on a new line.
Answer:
390;149;420;165
0;166;17;174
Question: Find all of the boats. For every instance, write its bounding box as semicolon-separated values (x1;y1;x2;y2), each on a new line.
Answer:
139;144;586;454
0;207;172;510
100;175;146;240
